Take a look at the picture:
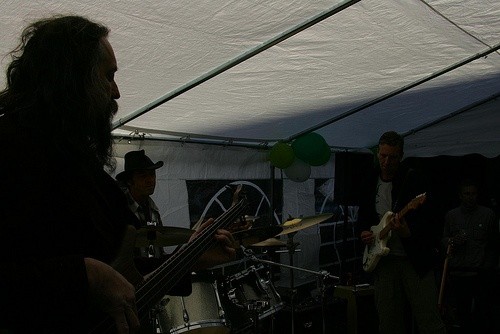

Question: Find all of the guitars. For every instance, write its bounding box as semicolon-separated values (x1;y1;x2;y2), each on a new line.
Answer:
82;190;251;334
361;192;428;274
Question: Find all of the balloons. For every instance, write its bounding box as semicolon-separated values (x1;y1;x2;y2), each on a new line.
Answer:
292;131;331;167
284;154;311;183
271;142;294;168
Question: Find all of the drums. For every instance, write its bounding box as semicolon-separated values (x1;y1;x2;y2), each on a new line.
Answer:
153;274;231;334
220;264;285;332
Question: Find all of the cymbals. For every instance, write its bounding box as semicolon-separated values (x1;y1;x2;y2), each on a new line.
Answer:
133;225;196;247
250;237;301;248
274;213;335;236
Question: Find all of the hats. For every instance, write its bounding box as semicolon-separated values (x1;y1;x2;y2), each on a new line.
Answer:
115;150;164;180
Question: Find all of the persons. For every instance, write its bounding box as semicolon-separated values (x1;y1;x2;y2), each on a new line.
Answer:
354;131;447;334
0;15;239;334
440;179;500;334
115;150;164;334
